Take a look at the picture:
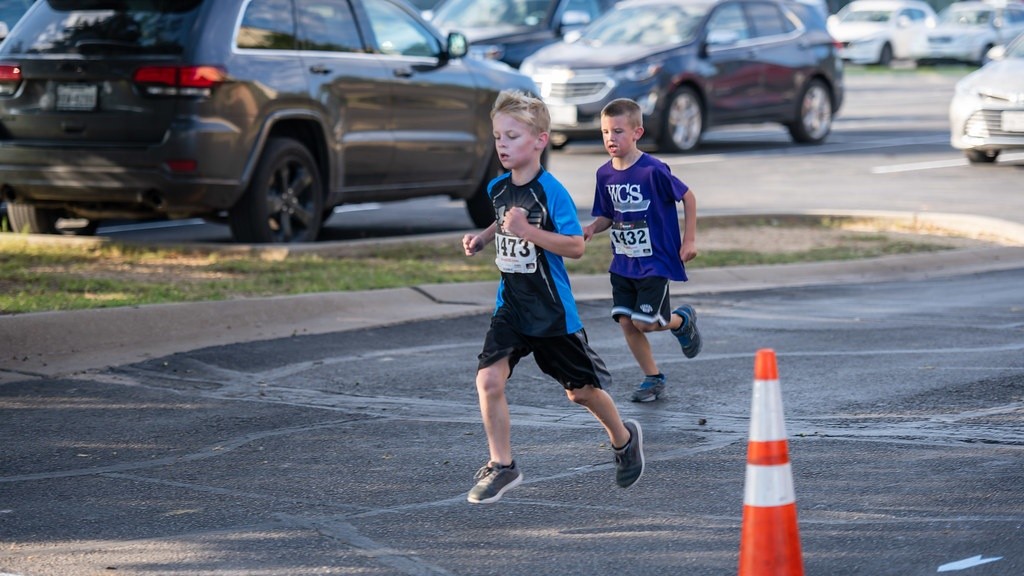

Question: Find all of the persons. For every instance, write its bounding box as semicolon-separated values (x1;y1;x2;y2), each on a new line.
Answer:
582;98;703;403
461;88;646;504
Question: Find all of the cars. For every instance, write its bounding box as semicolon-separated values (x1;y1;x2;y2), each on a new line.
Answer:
380;1;1023;163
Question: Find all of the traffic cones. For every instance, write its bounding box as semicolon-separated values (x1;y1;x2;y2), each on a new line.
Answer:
738;349;805;576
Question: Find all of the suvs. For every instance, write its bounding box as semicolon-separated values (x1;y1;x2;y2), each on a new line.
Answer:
0;1;549;247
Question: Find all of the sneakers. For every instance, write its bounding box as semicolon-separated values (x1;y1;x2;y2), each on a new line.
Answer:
671;304;702;358
467;459;524;503
610;419;645;489
630;374;668;402
